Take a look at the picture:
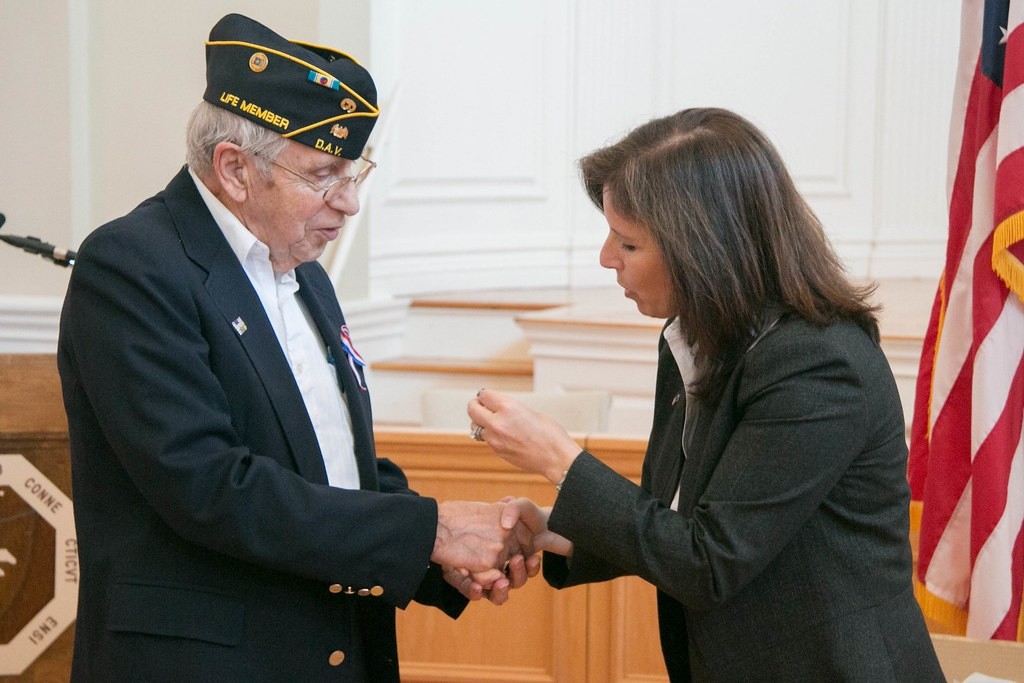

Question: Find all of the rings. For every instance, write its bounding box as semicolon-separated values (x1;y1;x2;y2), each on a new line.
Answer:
503;559;510;575
471;425;484;442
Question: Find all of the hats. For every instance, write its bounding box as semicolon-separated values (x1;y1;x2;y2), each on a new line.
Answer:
202;14;380;160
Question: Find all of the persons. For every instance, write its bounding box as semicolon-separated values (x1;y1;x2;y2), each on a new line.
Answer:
457;109;947;683
55;14;537;683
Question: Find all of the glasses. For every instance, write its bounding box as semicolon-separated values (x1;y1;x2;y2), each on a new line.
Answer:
230;138;377;202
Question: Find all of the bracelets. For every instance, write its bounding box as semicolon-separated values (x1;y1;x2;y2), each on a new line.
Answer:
556;470;568;493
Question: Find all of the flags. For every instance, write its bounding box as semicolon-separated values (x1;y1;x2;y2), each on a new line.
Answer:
908;0;1024;645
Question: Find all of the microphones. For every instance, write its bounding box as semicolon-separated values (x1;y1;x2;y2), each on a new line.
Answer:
1;236;77;267
